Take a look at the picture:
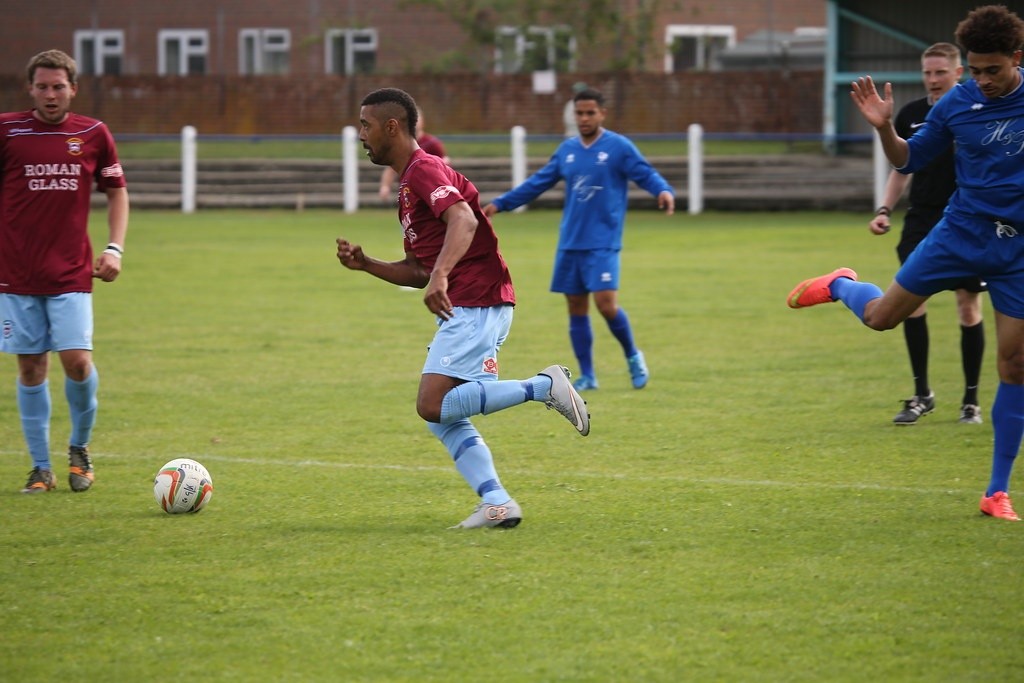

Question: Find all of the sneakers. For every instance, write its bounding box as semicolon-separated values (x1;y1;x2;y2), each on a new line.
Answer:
786;266;858;309
960;403;981;425
570;372;599;392
538;365;592;437
626;351;647;389
979;491;1022;521
20;465;55;494
70;445;93;492
894;392;934;425
448;498;522;531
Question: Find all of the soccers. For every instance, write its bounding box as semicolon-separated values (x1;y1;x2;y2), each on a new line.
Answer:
152;458;213;515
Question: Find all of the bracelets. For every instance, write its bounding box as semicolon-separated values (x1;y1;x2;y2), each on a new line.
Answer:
878;207;890;217
107;246;121;254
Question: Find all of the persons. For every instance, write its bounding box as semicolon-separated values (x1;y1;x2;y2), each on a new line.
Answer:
786;5;1024;521
482;89;674;391
380;106;450;291
869;43;986;426
0;49;129;495
335;87;592;530
